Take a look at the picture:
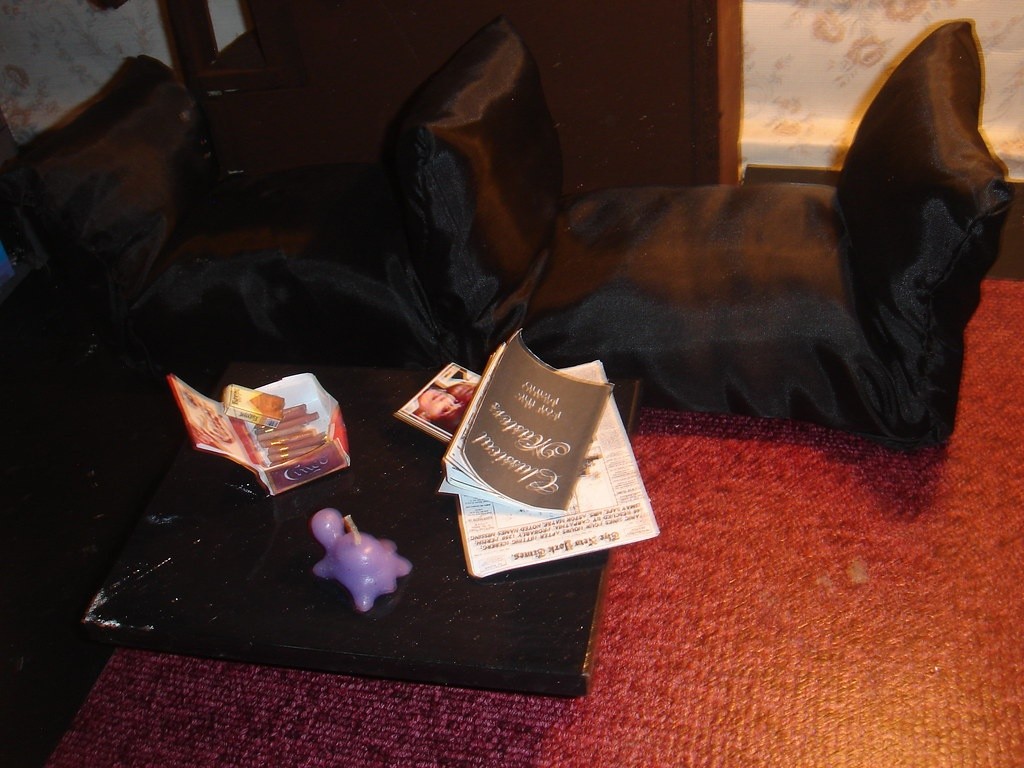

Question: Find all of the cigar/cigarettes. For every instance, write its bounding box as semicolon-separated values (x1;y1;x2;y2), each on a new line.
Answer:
254;404;328;468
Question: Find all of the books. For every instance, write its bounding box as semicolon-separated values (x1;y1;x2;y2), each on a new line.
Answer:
438;329;615;516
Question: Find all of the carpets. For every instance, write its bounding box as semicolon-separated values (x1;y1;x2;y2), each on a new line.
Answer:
44;279;1024;768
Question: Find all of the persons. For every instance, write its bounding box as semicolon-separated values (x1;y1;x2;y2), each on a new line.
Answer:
405;366;478;436
